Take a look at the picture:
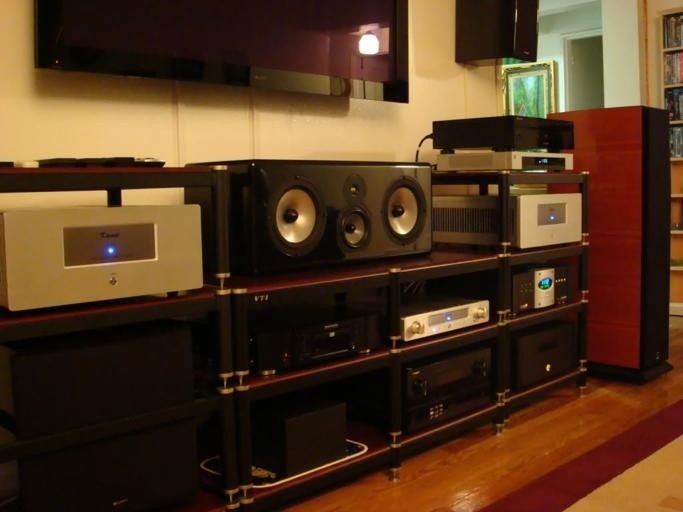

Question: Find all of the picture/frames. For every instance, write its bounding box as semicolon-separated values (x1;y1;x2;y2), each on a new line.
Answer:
500;60;556;153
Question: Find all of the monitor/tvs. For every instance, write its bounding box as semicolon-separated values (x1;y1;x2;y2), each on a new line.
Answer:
34;0;409;103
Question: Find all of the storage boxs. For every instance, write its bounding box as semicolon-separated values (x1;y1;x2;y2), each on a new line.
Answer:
14;430;199;512
514;322;577;390
259;400;347;478
2;323;196;442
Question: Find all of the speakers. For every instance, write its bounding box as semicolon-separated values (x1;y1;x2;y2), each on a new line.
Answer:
11;327;195;512
272;393;347;473
547;106;670;369
456;0;538;67
185;161;432;278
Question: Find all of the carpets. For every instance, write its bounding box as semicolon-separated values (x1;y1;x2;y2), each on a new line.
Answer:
475;394;682;512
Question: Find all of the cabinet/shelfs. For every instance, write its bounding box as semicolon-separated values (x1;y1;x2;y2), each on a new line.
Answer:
656;6;681;318
0;160;591;512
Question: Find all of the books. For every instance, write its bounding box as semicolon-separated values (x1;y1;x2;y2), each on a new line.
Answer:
660;12;682;159
